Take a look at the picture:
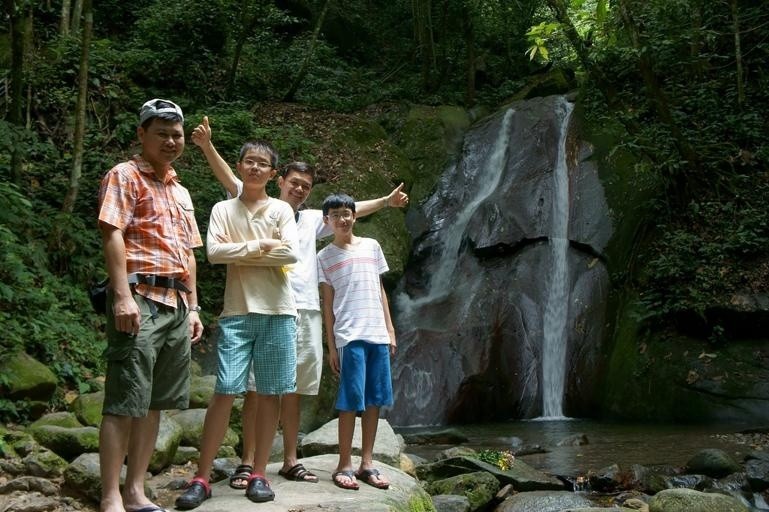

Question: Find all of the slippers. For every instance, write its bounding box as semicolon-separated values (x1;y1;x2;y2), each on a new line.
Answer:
332;471;359;490
354;468;389;489
230;465;253;489
279;463;319;482
128;506;168;512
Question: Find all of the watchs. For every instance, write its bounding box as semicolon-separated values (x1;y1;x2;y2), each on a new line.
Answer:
186;304;201;314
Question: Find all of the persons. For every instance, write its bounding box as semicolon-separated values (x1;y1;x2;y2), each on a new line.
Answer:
190;115;411;492
315;191;399;490
95;98;203;510
175;136;300;510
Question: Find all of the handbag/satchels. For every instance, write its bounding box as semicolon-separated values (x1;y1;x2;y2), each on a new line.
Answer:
88;278;111;314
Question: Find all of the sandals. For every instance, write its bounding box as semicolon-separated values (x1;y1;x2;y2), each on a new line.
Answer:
175;478;211;509
246;474;275;501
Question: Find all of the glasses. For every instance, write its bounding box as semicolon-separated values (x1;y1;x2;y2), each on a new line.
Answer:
242;158;272;168
325;212;352;219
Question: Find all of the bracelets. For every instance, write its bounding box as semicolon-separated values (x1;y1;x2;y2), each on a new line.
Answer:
381;195;389;211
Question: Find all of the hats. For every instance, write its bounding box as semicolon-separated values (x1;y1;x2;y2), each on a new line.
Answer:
140;99;184;126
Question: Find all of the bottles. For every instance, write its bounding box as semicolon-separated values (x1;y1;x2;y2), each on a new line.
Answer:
273;226;296;274
